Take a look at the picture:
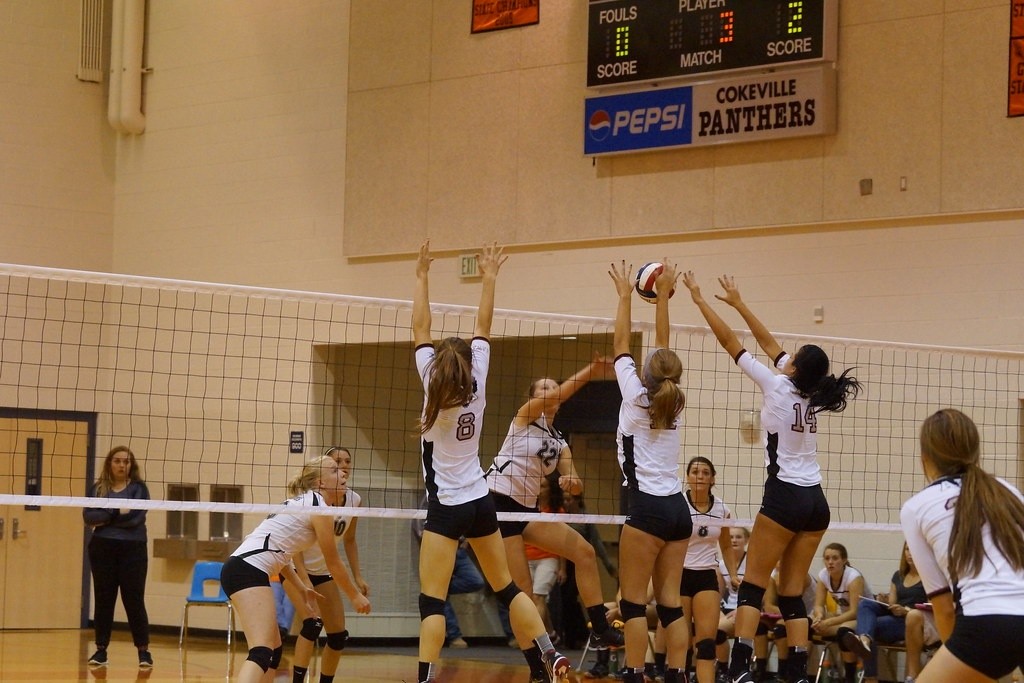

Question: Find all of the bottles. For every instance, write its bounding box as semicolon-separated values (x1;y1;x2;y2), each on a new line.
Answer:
821;660;832;683
607;647;619;677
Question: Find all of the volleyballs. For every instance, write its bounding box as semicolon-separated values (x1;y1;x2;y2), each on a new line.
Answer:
635;262;677;304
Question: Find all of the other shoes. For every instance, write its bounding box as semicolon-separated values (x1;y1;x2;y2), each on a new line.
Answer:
529;671;551;683
449;638;466;650
842;632;872;659
509;639;522;650
547;631;559;646
584;664;609;677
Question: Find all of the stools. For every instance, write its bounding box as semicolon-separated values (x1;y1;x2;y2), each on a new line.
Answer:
575;632;907;683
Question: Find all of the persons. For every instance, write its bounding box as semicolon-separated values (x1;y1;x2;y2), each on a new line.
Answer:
485;351;625;683
410;239;569;683
901;408;1024;683
521;470;619;651
682;456;742;683
414;494;485;649
82;446;154;666
221;455;371;683
584;527;941;683
608;256;693;683
682;269;862;683
278;446;369;683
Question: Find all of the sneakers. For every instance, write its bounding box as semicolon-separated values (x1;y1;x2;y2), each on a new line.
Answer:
537;653;568;682
138;667;153;678
138;650;153;667
589;621;625;650
610;664;866;683
89;666;105;679
88;650;108;664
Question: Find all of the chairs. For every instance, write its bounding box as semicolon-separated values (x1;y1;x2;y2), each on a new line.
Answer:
180;561;236;645
